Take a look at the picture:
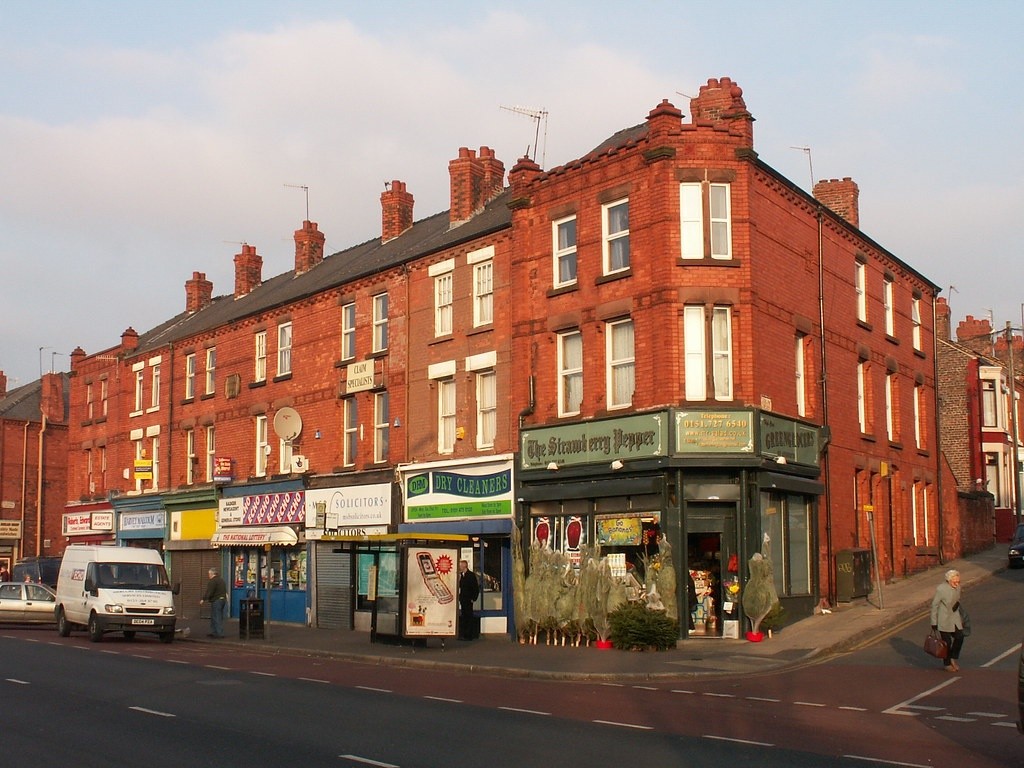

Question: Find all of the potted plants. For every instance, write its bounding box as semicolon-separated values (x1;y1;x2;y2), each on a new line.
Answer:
586;560;626;649
740;558;778;641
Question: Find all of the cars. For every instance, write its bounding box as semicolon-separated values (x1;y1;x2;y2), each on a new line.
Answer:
1;584;56;624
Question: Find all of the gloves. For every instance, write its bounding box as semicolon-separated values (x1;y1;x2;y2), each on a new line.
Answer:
952;601;960;613
931;625;938;632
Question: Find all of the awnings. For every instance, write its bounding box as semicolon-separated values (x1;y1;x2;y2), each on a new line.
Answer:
212;524;299;548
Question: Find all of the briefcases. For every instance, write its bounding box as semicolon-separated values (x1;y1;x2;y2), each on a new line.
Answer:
924;627;947;660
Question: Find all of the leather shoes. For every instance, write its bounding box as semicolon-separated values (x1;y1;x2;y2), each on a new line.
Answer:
943;664;957;673
950;658;960;672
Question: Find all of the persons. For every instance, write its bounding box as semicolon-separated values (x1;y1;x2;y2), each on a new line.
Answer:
930;570;966;672
198;567;229;638
456;559;480;640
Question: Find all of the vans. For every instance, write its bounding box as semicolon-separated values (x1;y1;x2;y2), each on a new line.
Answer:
53;545;181;643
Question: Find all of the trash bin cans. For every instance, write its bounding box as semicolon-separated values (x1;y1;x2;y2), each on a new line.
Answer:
239;598;264;638
